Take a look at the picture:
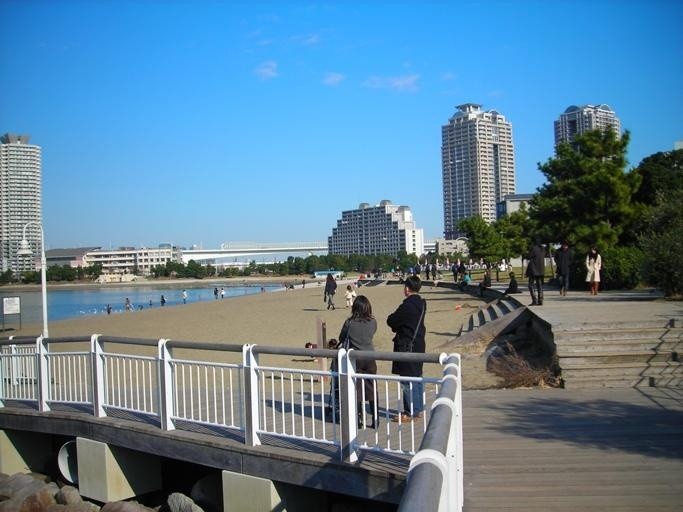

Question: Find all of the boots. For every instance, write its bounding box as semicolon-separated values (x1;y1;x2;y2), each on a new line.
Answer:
369;398;379;429
356;401;362;428
589;281;600;296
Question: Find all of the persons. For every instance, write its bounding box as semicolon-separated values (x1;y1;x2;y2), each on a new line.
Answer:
106;283;225;314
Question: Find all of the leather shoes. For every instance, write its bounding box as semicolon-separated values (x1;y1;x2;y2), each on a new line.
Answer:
529;299;537;306
538;298;544;305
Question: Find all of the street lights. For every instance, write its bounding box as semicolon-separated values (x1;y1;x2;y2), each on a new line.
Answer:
15;220;56;384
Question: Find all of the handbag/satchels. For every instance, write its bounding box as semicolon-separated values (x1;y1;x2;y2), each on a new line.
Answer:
342;338;349;349
324;291;326;302
392;335;414;353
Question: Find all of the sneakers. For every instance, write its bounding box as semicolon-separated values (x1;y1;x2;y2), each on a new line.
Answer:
392;411;423;422
